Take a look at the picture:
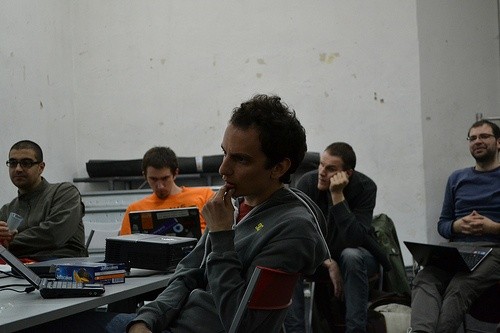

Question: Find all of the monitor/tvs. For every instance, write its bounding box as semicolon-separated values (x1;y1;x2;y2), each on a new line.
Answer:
127;206;202;243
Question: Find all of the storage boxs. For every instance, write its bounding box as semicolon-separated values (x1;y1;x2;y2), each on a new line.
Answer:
50;261;127;285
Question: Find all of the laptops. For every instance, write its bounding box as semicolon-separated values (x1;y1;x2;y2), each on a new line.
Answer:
0;244;104;298
403;240;492;272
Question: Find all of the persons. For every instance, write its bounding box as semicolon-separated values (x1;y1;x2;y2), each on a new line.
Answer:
0;140;88;259
281;143;377;333
32;96;327;333
119;146;216;236
411;120;500;333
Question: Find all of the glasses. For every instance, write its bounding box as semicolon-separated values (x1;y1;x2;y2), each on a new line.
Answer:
467;134;496;141
6;160;41;169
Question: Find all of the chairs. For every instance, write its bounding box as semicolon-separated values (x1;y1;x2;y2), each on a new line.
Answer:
227;265;303;333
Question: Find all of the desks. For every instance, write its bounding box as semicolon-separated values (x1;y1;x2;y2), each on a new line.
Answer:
0;272;171;333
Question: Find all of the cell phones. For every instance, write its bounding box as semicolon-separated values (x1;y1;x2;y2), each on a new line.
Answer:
7;212;24;231
